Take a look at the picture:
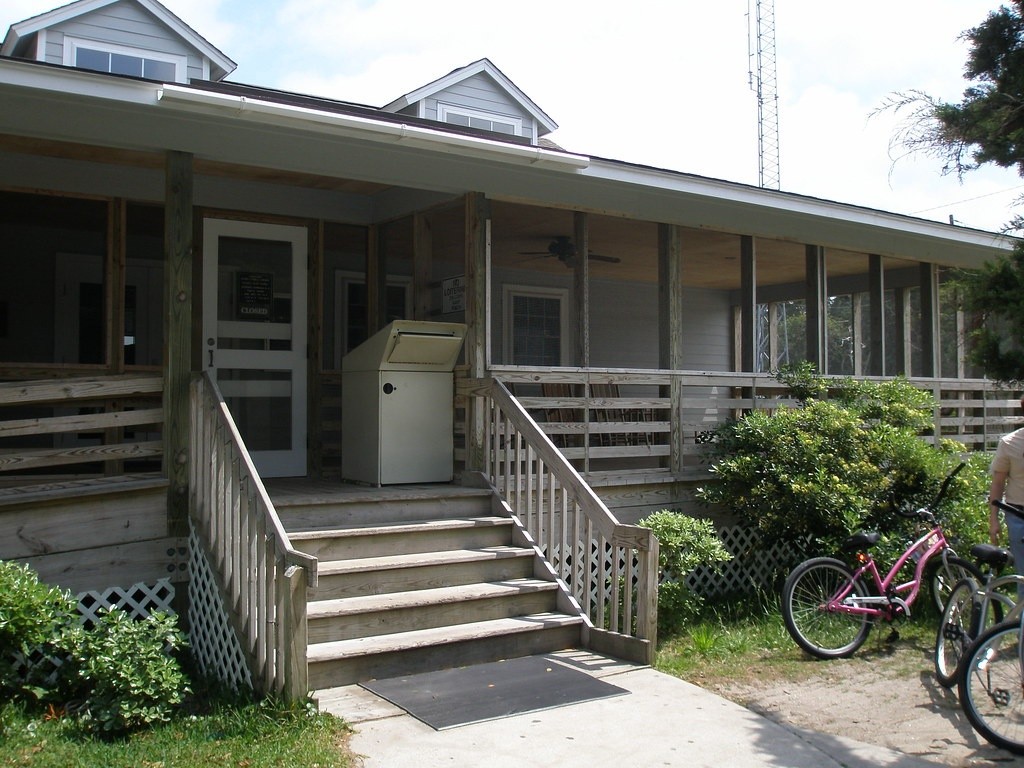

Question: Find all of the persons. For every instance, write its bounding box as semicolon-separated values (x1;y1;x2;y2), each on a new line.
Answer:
990;394;1024;700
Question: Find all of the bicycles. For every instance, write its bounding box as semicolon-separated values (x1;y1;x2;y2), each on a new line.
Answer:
782;463;1003;660
935;499;1024;761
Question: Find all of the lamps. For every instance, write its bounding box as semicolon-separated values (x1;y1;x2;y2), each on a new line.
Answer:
550;238;583;261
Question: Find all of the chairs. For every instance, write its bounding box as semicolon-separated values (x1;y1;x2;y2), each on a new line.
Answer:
502;382;657;448
